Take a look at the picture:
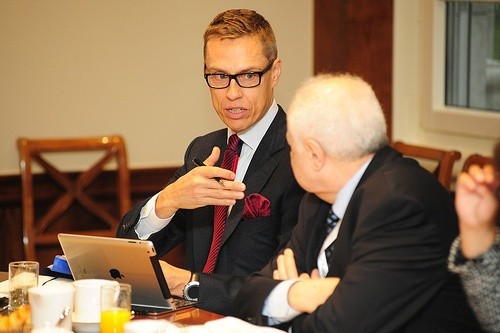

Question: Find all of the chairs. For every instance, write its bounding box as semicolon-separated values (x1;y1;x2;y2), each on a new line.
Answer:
17;137;132;265
392;141;462;192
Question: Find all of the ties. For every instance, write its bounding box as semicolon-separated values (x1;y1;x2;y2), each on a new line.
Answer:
203;134;244;274
317;209;339;272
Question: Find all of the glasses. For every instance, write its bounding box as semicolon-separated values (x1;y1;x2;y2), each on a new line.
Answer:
204;60;274;88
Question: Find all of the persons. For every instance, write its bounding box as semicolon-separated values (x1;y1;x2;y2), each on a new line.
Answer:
117;8;500;333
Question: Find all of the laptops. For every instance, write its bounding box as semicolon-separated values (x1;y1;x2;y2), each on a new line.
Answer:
56;233;198;316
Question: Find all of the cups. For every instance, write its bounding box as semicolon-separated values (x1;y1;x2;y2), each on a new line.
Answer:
73;279;120;323
29;284;74;327
101;283;132;333
8;261;39;316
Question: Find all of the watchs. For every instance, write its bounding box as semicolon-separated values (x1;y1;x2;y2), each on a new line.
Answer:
181;271;200;305
